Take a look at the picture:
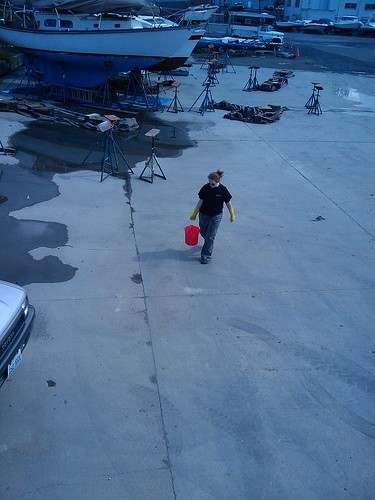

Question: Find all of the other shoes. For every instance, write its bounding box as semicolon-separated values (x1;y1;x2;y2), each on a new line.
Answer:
201;257;208;264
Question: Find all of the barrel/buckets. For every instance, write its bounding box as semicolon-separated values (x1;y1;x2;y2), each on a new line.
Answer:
184;217;201;245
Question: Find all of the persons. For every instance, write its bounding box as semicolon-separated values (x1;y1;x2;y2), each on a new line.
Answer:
187;169;235;265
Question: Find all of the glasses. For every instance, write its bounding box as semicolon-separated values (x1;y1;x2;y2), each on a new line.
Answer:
209;181;218;186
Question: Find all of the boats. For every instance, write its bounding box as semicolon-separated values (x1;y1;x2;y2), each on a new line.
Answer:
0;0;375;90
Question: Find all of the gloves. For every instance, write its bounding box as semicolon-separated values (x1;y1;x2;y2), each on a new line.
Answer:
190;208;199;220
229;208;235;222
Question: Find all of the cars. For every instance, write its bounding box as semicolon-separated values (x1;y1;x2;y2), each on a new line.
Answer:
0;279;38;386
